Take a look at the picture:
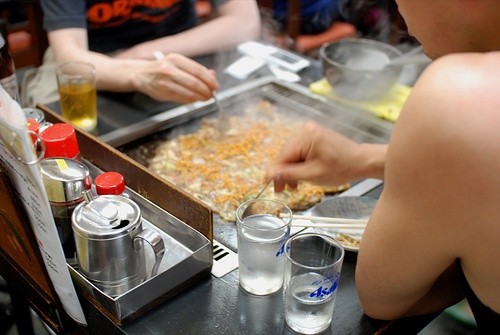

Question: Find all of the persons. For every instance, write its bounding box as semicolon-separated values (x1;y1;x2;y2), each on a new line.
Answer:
264;0;500;335
39;0;262;102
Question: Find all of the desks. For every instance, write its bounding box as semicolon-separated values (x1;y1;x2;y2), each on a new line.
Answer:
0;35;465;335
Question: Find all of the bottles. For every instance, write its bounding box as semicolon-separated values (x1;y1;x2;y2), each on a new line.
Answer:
0;35;21;103
39;123;82;163
9;31;38;94
95;172;132;199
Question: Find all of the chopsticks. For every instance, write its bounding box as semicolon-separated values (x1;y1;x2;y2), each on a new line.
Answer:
278;212;368;229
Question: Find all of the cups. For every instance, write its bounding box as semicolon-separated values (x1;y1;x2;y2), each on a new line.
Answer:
71;188;166;297
234;198;293;299
55;60;98;133
38;156;92;268
282;232;345;335
22;106;46;124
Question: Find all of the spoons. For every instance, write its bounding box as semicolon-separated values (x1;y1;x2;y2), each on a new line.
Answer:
241;178;273;219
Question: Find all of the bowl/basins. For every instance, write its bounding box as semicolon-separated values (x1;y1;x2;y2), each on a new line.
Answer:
310;196;377;252
319;38;406;102
